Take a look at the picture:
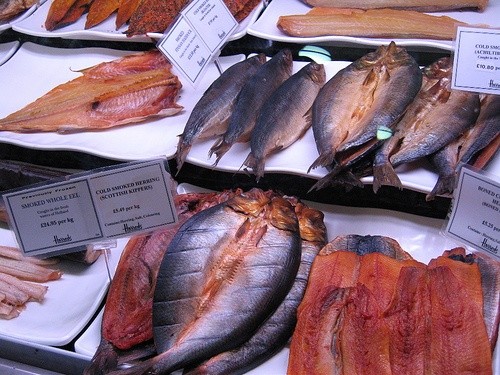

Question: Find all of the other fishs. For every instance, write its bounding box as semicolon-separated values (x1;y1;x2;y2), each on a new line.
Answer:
166;43;500;202
0;0;261;38
0;50;183;134
0;186;500;375
277;0;492;43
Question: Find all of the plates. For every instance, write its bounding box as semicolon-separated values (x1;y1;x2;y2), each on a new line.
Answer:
11;0;265;42
247;0;500;51
75;184;500;375
0;0;46;41
0;226;130;346
0;41;246;160
184;53;500;198
